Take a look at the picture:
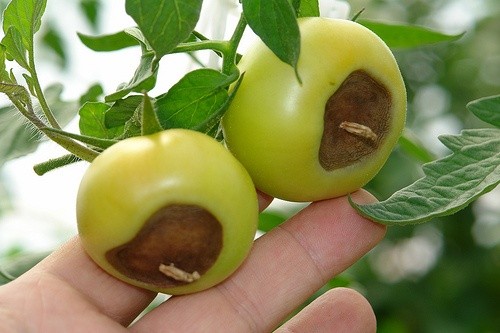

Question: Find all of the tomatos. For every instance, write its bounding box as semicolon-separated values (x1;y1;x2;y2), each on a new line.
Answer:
221;16;411;204
75;128;261;296
